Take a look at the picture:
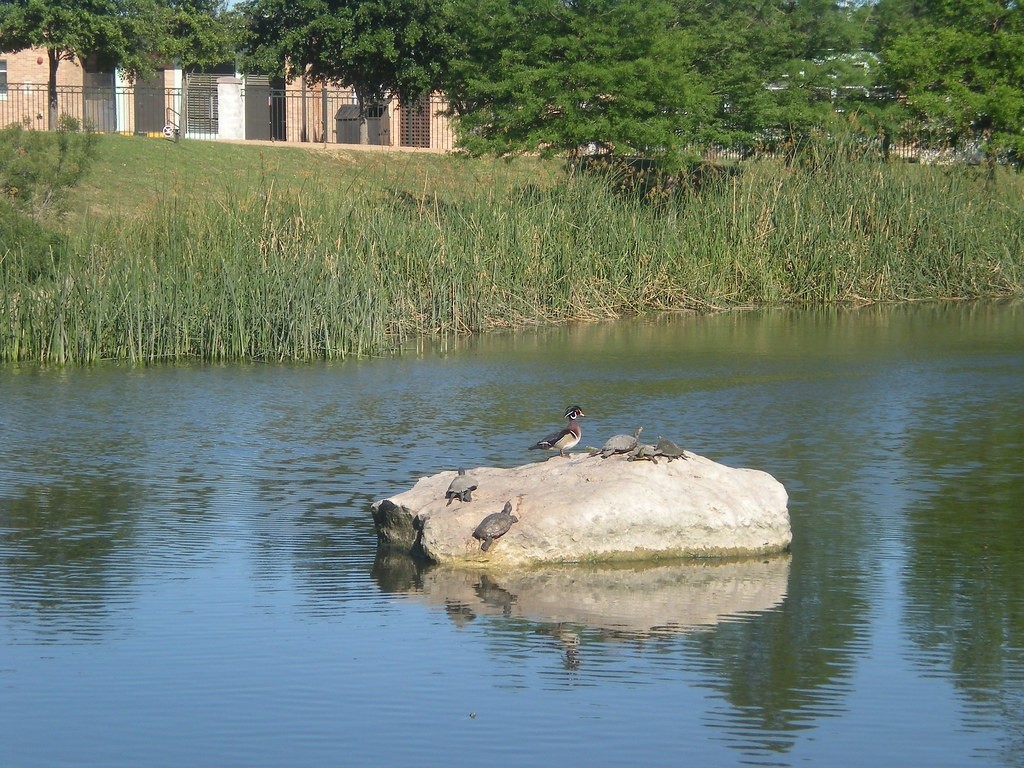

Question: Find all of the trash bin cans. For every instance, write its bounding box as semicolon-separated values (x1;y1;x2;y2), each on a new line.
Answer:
334;104;390;145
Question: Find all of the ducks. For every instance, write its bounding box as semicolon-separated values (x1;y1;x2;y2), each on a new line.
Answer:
527;406;586;458
